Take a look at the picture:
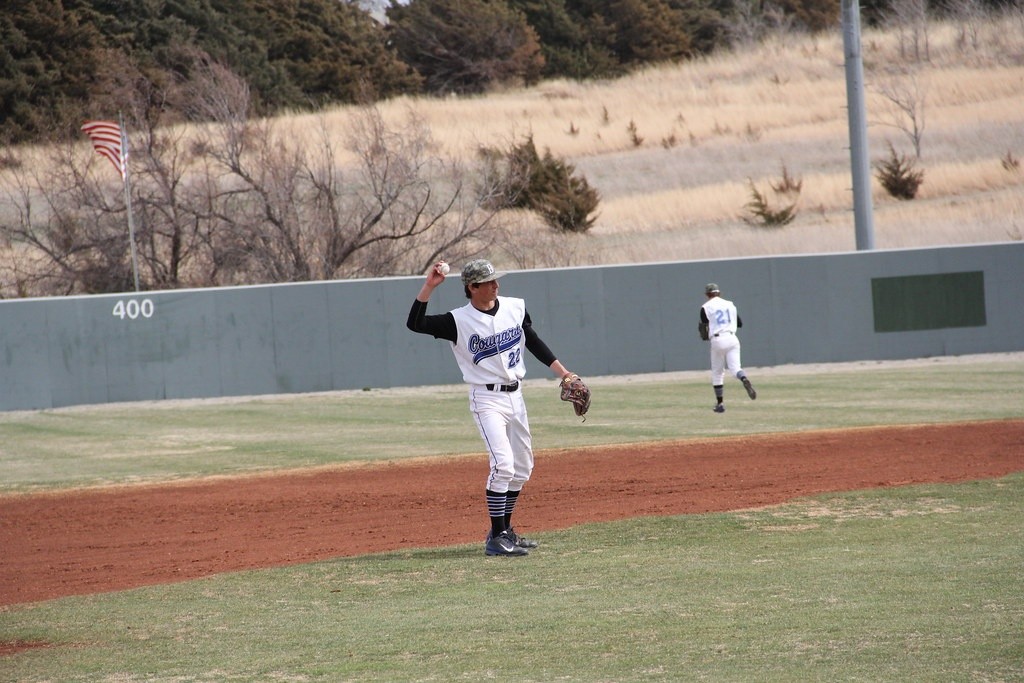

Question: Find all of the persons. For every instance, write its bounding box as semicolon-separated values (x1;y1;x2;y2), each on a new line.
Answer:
698;282;756;412
405;258;591;556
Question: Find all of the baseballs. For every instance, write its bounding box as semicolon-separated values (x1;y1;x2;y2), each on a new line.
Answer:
438;263;450;275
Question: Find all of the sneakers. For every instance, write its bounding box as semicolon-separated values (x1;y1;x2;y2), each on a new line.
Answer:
713;403;724;412
485;531;528;555
486;526;538;549
743;379;756;400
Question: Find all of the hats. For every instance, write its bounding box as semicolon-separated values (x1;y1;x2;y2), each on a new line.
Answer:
462;259;508;285
704;283;720;293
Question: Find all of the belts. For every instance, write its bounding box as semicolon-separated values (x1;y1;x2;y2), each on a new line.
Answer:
486;379;522;392
714;331;732;336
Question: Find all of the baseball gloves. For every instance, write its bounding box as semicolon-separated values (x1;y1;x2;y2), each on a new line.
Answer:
561;372;591;416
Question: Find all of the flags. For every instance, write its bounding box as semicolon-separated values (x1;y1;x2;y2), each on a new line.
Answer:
81;119;128;182
